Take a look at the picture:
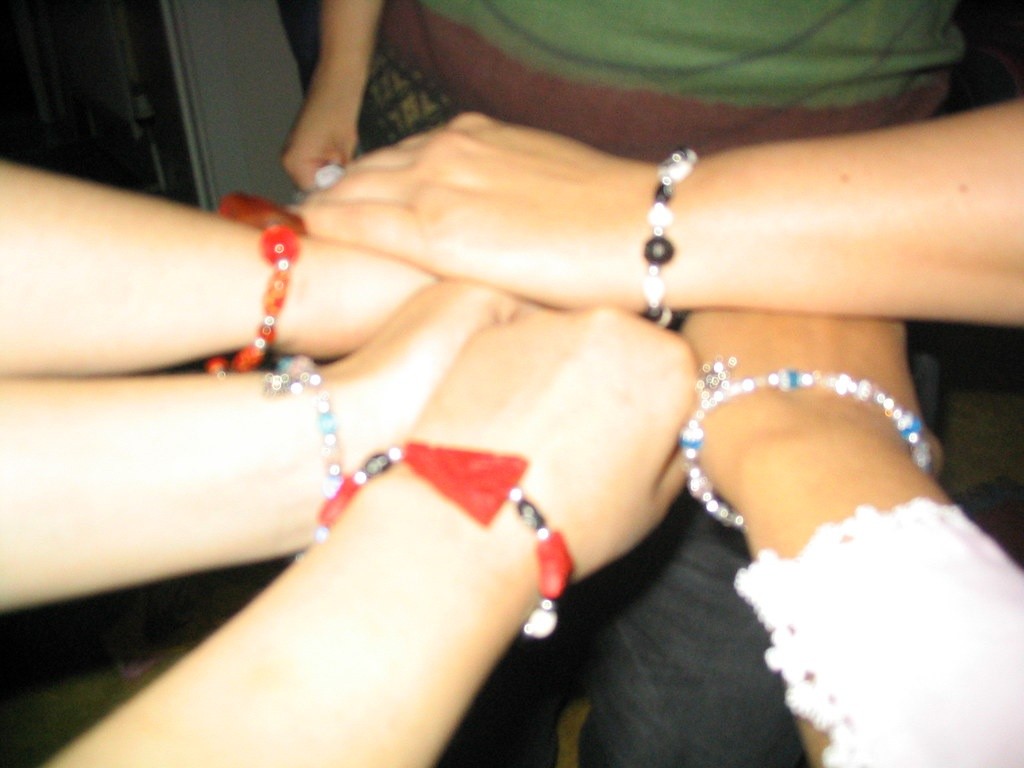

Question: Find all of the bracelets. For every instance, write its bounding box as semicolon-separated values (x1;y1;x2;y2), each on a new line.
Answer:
680;366;933;528
315;442;570;638
272;356;343;497
206;194;299;373
642;147;698;325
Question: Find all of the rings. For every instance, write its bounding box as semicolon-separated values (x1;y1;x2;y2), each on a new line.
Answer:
316;164;343;191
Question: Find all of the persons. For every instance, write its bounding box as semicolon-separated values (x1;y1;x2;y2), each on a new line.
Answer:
285;1;966;768
0;101;1024;768
